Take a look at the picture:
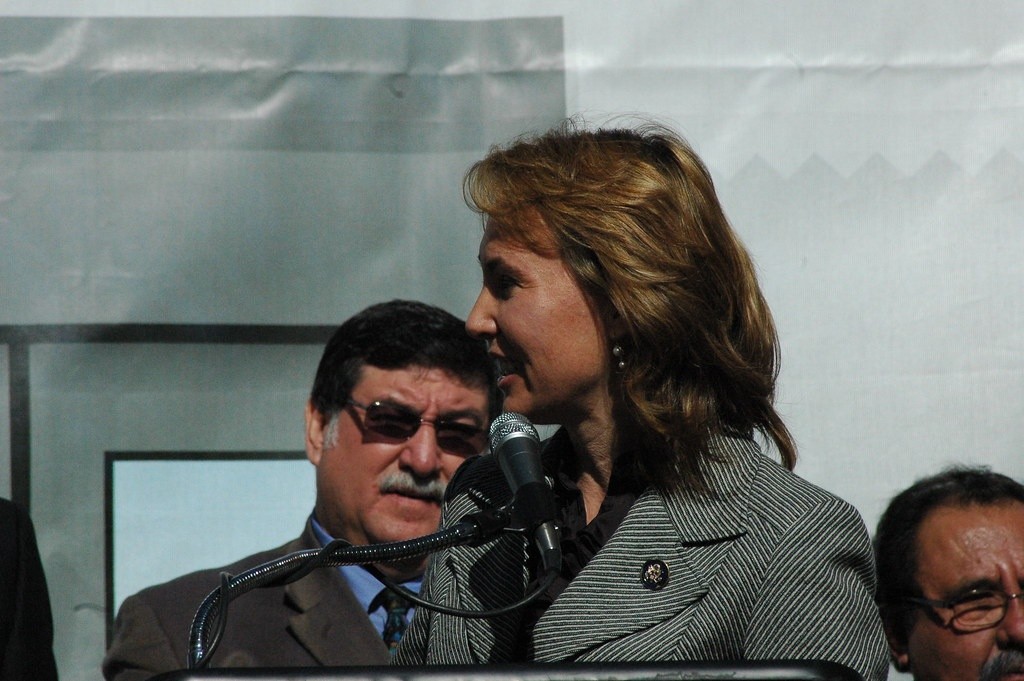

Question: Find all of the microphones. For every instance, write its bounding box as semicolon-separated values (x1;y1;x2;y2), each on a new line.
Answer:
488;413;561;579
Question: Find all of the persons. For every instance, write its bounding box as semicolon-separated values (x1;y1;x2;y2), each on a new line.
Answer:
103;300;504;681
389;130;887;681
0;498;60;681
874;467;1024;681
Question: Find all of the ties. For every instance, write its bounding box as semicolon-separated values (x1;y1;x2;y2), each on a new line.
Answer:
376;584;419;657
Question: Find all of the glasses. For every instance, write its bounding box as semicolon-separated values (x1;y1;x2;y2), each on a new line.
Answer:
903;589;1024;626
337;394;490;457
